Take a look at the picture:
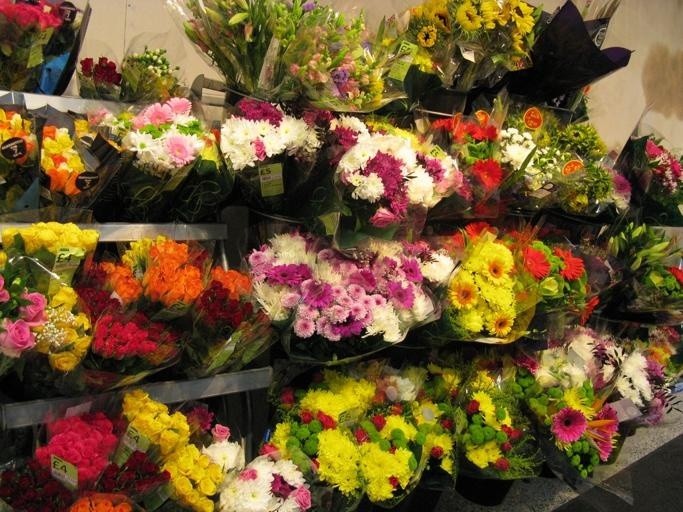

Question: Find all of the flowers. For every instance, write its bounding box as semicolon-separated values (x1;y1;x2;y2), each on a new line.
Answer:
2;1;683;510
